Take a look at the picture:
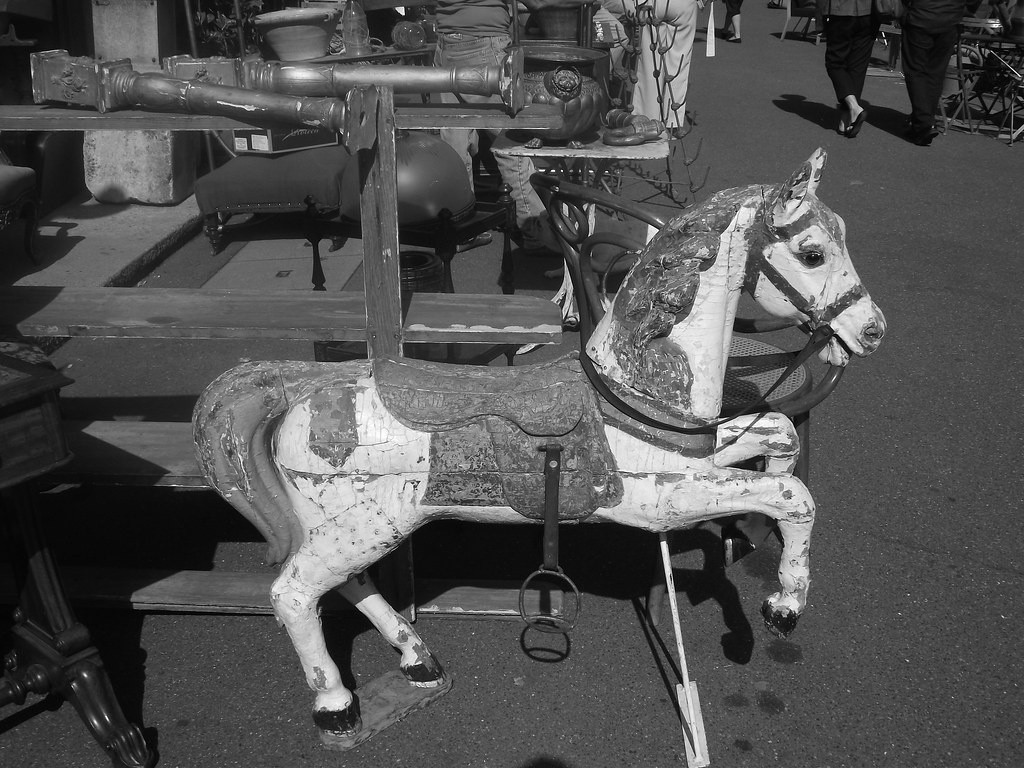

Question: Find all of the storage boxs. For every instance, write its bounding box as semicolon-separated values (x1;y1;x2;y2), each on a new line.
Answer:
232;128;339;153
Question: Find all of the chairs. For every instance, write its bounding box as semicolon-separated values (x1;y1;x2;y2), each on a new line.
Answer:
780;0;822;46
530;173;851;486
935;18;1024;147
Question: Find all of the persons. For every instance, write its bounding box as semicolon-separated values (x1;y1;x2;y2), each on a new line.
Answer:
901;0;983;146
816;0;883;137
722;0;743;43
433;0;707;270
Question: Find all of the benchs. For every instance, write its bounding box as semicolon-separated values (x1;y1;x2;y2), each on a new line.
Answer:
195;144;347;252
0;166;37;264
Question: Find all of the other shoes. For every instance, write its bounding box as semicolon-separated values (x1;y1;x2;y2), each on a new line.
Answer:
915;124;939;146
726;36;742;43
718;28;730;35
543;255;564;277
457;232;491;252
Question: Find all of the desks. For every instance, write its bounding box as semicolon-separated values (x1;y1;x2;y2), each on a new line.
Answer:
960;35;1024;139
0;352;159;768
490;130;670;355
298;184;514;294
303;45;435;106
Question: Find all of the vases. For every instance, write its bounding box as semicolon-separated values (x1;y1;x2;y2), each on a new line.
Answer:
248;8;340;62
399;251;443;294
517;0;601;39
503;45;608;148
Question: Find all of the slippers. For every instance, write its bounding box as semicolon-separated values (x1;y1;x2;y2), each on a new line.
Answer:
836;119;845;134
847;110;867;138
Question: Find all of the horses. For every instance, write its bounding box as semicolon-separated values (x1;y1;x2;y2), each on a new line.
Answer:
189;145;890;740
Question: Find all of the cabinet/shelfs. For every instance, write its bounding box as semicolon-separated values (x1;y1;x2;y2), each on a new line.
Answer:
0;103;561;620
